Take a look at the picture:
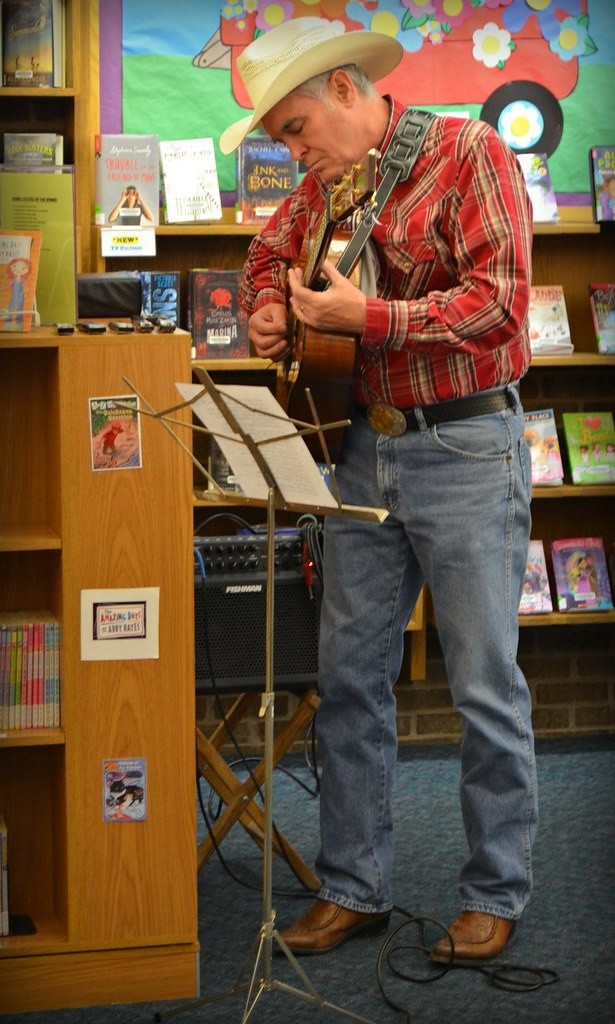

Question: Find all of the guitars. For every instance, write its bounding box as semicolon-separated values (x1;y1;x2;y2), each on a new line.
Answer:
275;148;382;465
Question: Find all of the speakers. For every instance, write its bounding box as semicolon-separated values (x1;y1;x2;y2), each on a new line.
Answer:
192;531;325;695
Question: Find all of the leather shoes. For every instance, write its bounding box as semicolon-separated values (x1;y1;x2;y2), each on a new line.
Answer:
279;899;390;953
432;911;515;963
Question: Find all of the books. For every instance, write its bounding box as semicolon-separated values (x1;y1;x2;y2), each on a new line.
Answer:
523;408;564;488
235;134;300;228
2;132;65;176
514;152;562;223
517;540;552;614
589;144;615;225
552;537;614;613
138;268;252;359
159;137;222;224
0;608;62;732
562;411;615;485
95;135;161;227
1;170;79;332
0;811;10;936
0;1;69;91
525;284;574;356
588;282;615;356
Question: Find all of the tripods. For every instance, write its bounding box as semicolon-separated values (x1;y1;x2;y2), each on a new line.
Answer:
115;363;390;1024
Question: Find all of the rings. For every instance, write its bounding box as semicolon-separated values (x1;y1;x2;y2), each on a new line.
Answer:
301;306;303;312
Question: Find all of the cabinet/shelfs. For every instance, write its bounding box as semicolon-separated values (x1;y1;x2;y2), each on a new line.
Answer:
0;0;615;1009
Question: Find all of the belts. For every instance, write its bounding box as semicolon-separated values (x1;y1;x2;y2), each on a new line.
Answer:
354;391;512;437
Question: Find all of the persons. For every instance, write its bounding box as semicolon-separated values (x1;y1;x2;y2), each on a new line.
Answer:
99;423;129;454
216;16;536;964
108;185;154;225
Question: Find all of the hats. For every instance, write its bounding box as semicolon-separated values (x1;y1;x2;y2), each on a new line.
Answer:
218;16;403;154
